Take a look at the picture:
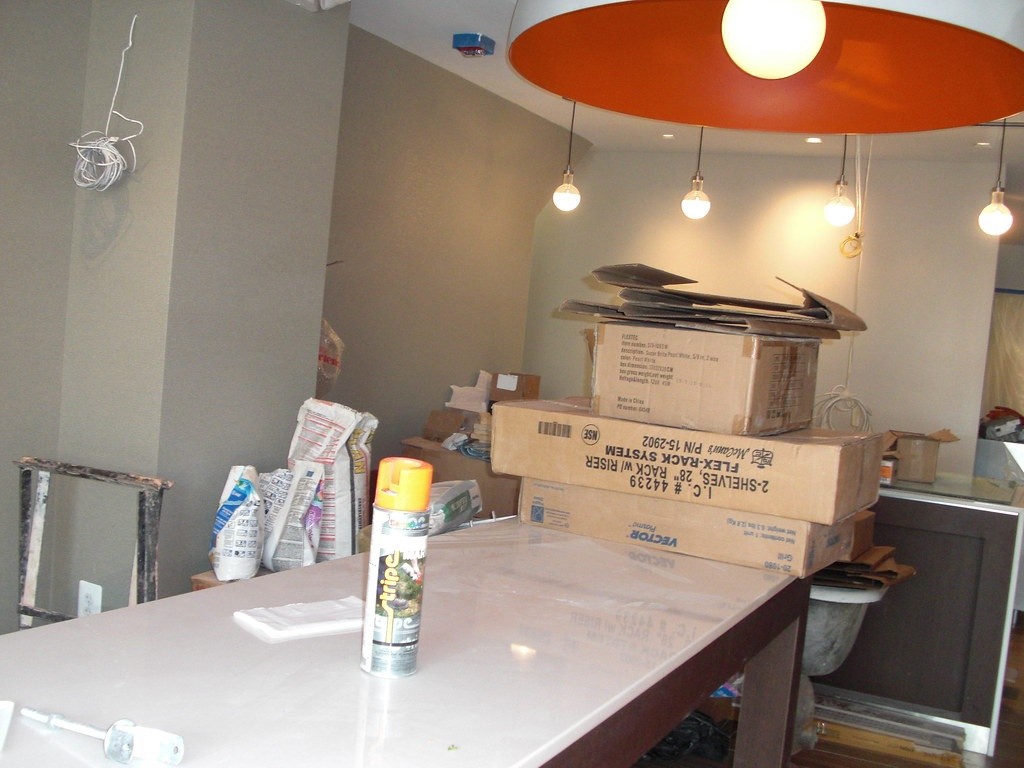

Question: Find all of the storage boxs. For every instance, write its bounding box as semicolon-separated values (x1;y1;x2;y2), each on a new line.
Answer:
839;511;876;563
489;372;540;403
590;318;823;434
491;393;883;526
402;441;521;521
518;478;855;580
883;429;961;483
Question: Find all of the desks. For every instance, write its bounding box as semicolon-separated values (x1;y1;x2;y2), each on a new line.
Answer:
0;518;812;768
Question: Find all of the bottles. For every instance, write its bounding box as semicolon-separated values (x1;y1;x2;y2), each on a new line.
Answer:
722;672;817;757
361;457;433;678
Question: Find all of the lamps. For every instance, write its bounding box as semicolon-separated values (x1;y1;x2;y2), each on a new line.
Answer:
552;101;581;212
825;134;855;226
722;0;826;81
681;122;711;219
977;119;1013;235
